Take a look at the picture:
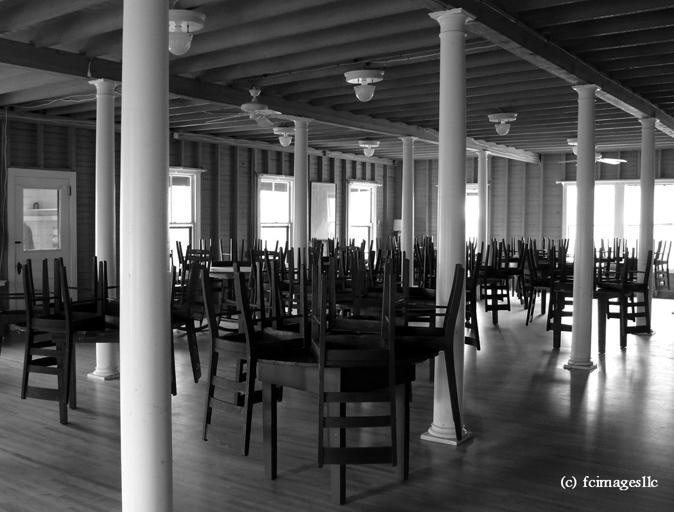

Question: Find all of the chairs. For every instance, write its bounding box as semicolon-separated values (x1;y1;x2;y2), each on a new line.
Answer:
9;229;673;503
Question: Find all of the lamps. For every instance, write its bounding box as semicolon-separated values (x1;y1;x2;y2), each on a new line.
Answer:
271;124;299;147
566;136;596;156
168;7;206;56
342;63;384;103
357;139;381;159
487;111;519;137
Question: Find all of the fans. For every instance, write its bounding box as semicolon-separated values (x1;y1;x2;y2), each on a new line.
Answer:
555;152;628;168
201;84;283;131
